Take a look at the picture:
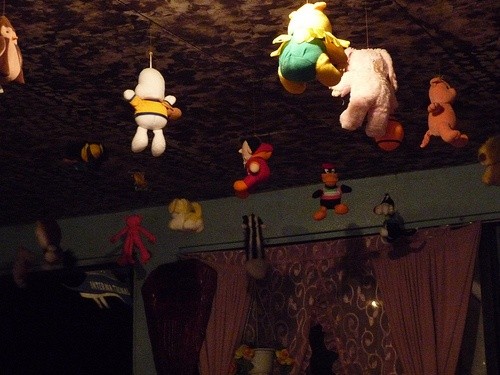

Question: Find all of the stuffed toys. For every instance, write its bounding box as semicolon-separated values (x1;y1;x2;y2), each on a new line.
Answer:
420;77;468;148
311;163;352;221
0;15;24;93
329;47;398;138
133;172;153;192
34;217;62;262
241;214;268;279
373;193;418;243
123;67;182;157
110;215;156;265
167;198;204;233
478;135;500;187
81;143;103;163
11;247;32;281
233;137;273;198
270;2;350;94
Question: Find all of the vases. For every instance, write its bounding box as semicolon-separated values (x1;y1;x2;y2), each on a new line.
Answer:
251;348;274;375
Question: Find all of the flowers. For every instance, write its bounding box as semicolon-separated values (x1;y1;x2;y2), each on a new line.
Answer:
235;343;292;375
